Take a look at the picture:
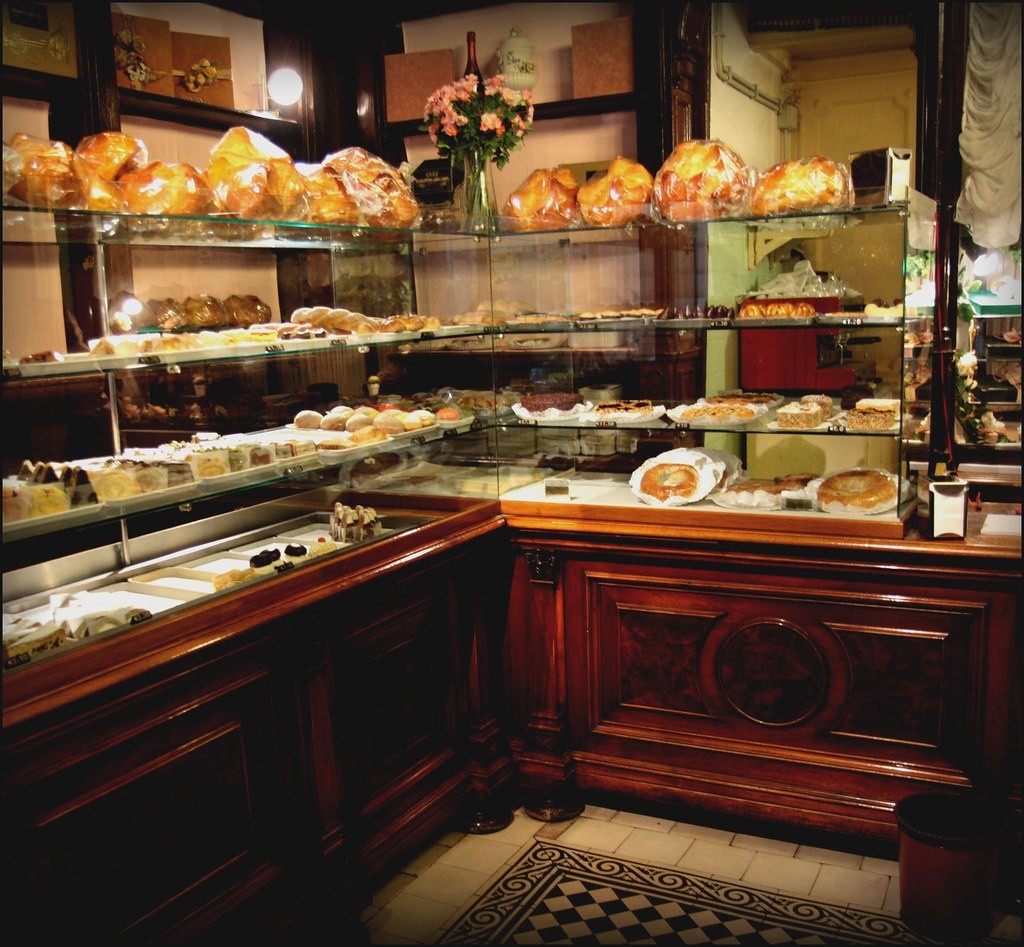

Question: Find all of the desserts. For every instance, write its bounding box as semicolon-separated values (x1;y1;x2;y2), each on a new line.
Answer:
214;537;335;594
2;441;273;520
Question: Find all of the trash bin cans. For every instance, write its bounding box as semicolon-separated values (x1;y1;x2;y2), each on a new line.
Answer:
893;792;1007;942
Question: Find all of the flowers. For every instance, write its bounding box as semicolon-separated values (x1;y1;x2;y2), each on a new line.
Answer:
949;243;1017;446
421;74;536;172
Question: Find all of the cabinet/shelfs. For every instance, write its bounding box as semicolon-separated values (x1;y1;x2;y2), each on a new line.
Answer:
0;482;514;947
490;205;921;542
0;196;502;705
906;335;1023;500
505;502;1024;862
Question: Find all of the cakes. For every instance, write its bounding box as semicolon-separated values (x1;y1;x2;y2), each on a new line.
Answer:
521;394;900;426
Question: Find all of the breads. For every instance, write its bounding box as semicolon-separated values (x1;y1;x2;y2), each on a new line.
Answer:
2;127;419;231
503;140;844;231
109;289;817;351
817;470;896;508
294;406;436;450
641;463;698;498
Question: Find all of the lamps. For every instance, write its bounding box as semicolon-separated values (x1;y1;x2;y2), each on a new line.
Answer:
109;290;143;322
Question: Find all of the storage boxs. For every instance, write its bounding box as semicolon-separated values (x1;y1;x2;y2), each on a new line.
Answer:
570;15;635;101
383;48;456;124
0;0;238;115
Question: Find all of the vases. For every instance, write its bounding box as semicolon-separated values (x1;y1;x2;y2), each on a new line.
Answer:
459;145;499;236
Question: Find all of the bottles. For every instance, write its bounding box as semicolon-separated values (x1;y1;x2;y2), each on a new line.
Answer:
463;32;484;105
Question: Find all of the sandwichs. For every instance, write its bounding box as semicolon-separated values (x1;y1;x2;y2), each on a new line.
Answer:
330;501;381;541
